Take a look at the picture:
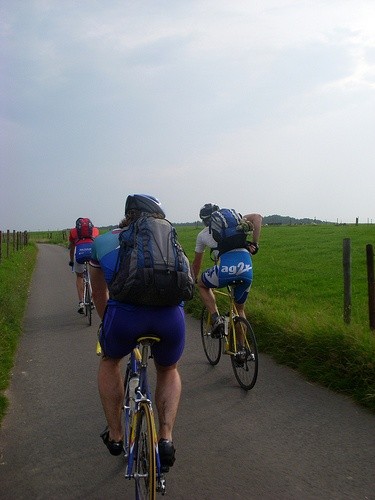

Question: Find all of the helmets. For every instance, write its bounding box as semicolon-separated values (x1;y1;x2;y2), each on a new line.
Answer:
199;203;219;221
125;194;166;219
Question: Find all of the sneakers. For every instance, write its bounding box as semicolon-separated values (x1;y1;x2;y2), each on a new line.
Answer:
90;302;95;308
158;437;176;467
210;316;225;339
76;301;85;313
100;425;124;456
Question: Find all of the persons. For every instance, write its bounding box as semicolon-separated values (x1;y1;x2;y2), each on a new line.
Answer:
89;193;186;465
192;203;262;367
69;218;99;313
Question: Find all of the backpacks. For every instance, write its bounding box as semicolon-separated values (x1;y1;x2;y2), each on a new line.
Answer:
76;218;99;243
108;217;195;311
209;209;254;262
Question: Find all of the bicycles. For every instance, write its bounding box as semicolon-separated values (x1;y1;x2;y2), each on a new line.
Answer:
96;323;169;500
70;258;95;325
193;277;259;390
